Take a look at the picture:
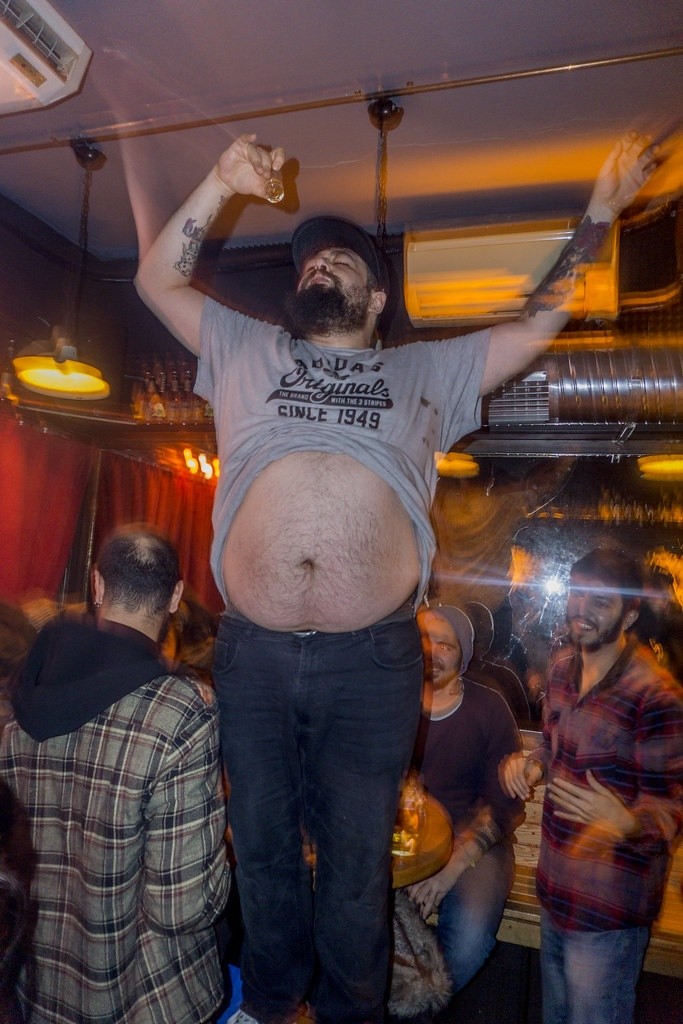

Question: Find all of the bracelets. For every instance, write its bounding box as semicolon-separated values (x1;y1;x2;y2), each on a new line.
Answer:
461;845;475;869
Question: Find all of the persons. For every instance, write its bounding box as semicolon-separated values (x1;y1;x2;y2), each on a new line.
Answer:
134;126;666;1023
0;523;683;1024
420;454;581;728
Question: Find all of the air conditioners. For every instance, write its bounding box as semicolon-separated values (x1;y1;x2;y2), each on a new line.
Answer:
0;0;94;119
397;215;619;326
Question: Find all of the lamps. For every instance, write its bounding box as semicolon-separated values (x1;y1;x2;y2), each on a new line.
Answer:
12;144;111;399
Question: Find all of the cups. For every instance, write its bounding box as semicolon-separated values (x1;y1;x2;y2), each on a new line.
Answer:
264;171;284;203
391;791;429;857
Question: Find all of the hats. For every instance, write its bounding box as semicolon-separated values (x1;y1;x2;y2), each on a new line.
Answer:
467;601;494;659
433;604;476;677
474;457;494;474
292;214;391;296
